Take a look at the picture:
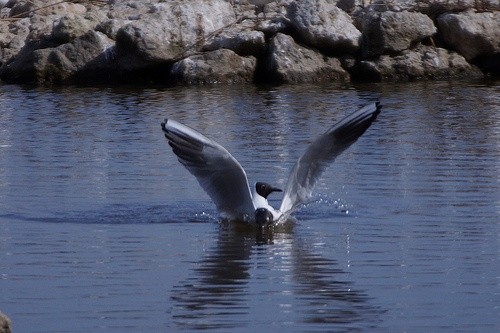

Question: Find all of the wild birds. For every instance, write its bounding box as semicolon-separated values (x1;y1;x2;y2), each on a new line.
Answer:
161;99;382;229
